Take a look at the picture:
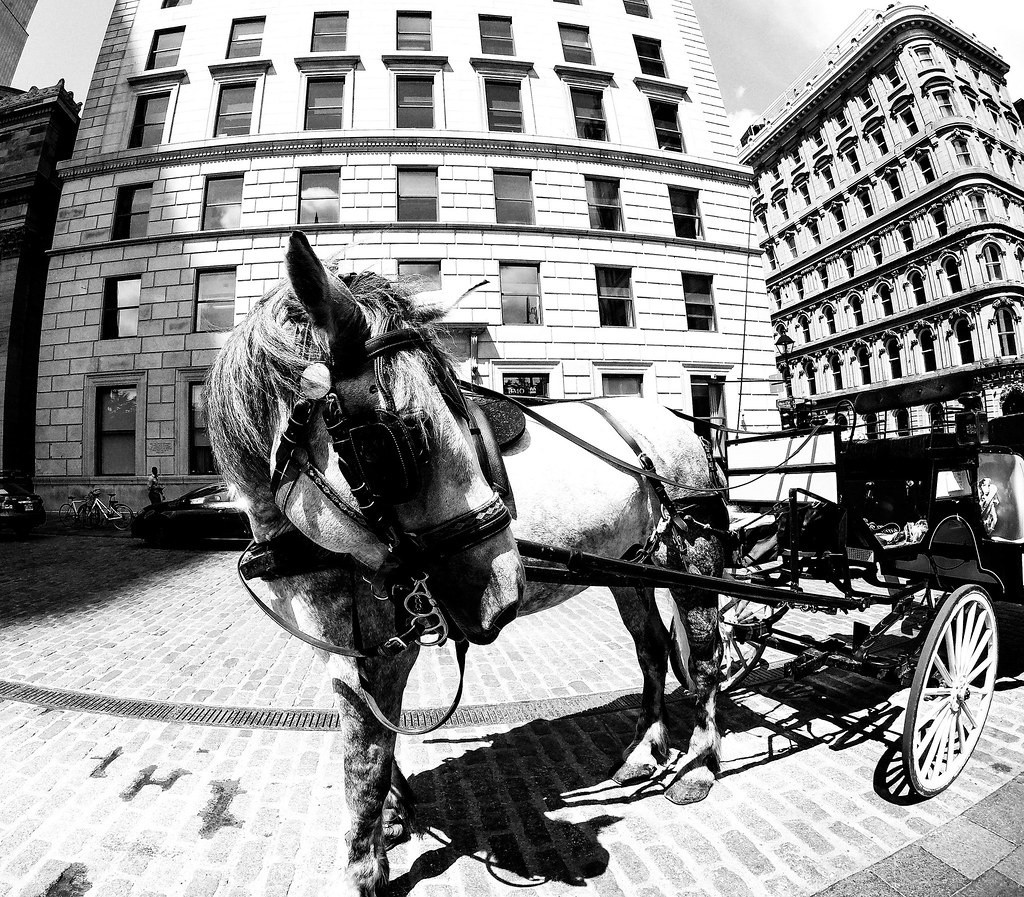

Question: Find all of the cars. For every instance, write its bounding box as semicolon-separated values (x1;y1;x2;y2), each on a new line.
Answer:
19;487;46;530
128;480;253;547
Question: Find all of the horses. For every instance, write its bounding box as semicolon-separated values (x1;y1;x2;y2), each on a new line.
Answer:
205;232;735;897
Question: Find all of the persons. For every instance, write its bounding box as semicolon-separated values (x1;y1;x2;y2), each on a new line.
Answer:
146;467;166;505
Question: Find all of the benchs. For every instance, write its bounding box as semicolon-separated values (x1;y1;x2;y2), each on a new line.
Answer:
832;433;981;571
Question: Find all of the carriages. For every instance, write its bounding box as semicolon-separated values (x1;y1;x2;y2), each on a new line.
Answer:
205;225;1024;897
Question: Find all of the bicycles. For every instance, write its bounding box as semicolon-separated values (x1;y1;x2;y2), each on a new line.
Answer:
58;486;134;533
